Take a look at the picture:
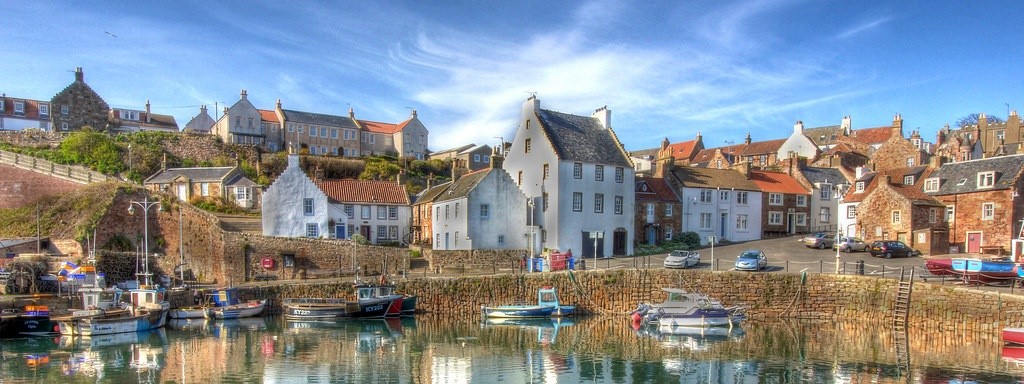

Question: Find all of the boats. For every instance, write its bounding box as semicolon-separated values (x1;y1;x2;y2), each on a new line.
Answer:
628;290;741;328
657;302;748;326
280;284;420;319
1000;344;1024;366
923;258;953;275
1001;327;1024;346
0;281;268;337
536;289;577;315
950;257;1017;277
484;305;559;317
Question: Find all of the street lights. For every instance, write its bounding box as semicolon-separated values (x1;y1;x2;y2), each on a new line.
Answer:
127;196;168;283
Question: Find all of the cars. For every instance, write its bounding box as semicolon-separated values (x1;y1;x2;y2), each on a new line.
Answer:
735;250;768;272
664;251;700;268
832;236;870;254
801;233;835;250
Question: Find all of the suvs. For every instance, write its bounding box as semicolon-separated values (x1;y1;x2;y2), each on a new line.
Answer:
869;240;914;258
